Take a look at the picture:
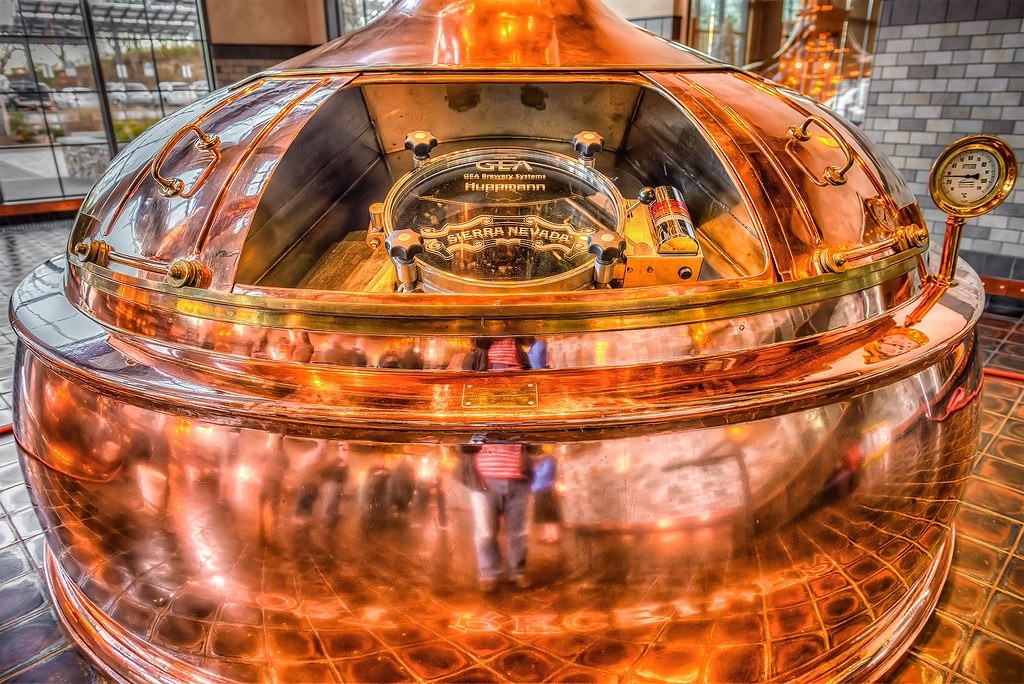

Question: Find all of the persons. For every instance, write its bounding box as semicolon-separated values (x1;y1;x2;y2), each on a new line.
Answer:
241;328;425;369
449;329;558;593
256;433;417;549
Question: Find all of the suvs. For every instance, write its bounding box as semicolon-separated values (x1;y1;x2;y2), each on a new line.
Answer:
1;80;51;114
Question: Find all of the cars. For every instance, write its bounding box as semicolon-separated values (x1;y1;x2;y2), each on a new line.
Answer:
50;87;100;109
101;80;153;107
191;80;208;97
151;81;197;105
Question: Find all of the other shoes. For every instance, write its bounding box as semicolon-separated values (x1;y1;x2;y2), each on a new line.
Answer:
507;574;534;589
480;572;505;592
